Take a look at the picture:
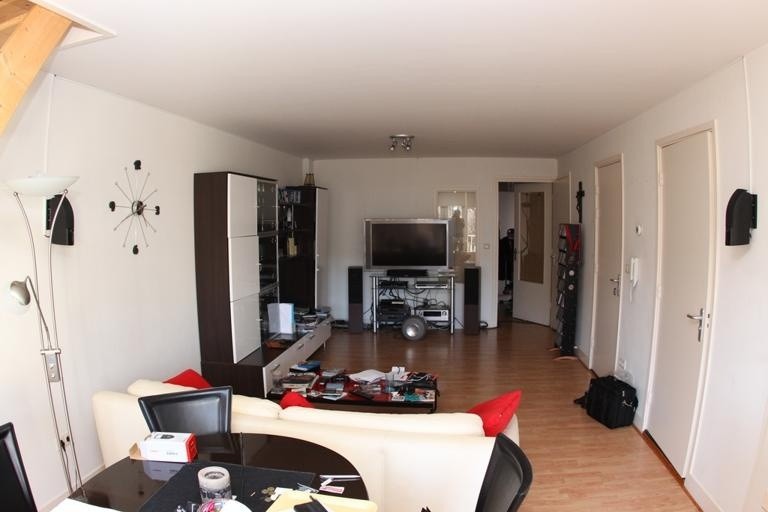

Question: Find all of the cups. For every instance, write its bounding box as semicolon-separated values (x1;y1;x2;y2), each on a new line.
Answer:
384;373;395;395
197;464;236;512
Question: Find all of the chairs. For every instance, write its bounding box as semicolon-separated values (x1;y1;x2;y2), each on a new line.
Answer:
1;422;41;512
474;433;534;511
139;383;232;435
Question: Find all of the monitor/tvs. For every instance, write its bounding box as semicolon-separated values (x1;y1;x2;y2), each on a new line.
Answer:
363;219;454;277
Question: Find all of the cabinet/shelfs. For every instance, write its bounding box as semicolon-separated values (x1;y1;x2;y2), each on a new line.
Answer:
545;223;583;362
368;273;457;335
192;171;333;398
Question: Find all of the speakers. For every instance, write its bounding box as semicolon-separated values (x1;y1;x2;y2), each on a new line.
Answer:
348;266;363;334
464;266;481;336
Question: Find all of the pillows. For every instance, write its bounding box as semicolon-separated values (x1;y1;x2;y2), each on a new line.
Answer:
468;387;524;437
161;367;216;394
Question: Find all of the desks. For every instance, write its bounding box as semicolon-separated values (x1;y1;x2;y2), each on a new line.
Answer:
50;432;373;511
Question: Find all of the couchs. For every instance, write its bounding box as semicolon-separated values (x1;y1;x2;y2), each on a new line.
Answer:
91;369;521;512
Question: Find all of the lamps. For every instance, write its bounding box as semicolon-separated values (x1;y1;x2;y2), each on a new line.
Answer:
386;133;416;153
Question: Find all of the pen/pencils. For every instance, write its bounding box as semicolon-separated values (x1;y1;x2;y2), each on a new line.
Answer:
320;474;361;479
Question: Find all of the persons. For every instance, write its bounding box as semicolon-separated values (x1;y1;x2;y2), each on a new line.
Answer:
500;228;514;295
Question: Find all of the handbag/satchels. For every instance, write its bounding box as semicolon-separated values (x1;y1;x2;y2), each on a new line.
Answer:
586;374;639;430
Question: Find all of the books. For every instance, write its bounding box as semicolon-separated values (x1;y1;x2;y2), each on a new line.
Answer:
554;224;567;350
295;308;329;333
271;359;437;402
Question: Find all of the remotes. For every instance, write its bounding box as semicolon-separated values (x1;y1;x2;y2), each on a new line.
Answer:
350;389;373;400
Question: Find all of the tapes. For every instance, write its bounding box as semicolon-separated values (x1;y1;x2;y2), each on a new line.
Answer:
198;466;230;490
